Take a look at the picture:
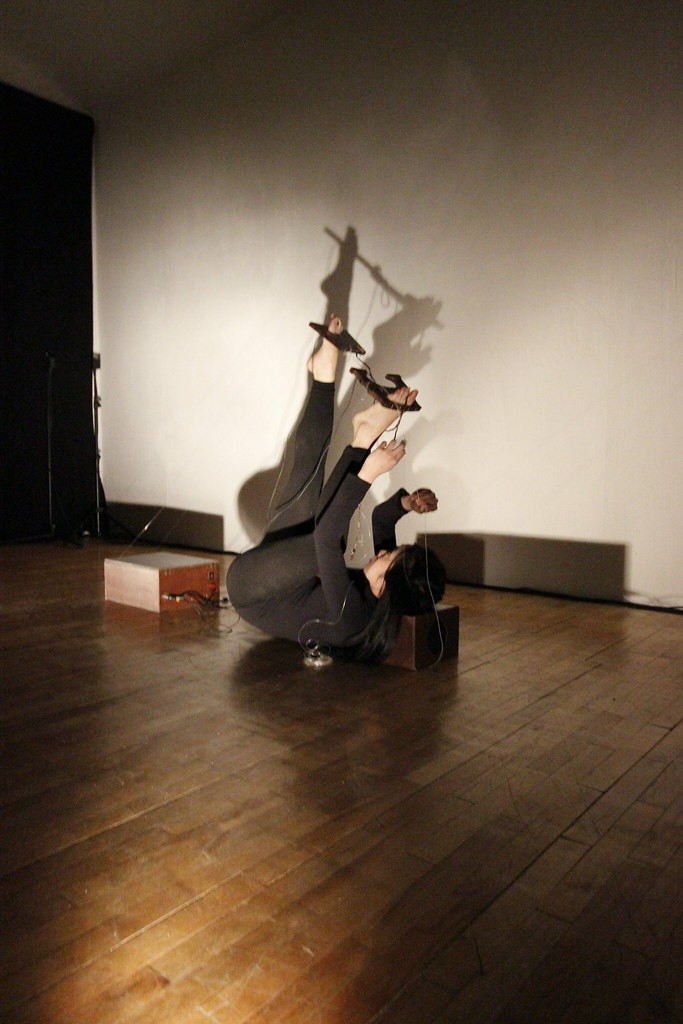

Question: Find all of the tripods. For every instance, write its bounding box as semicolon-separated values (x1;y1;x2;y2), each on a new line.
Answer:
68;359;143;546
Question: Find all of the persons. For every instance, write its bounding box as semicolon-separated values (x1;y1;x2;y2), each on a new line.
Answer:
226;312;446;663
